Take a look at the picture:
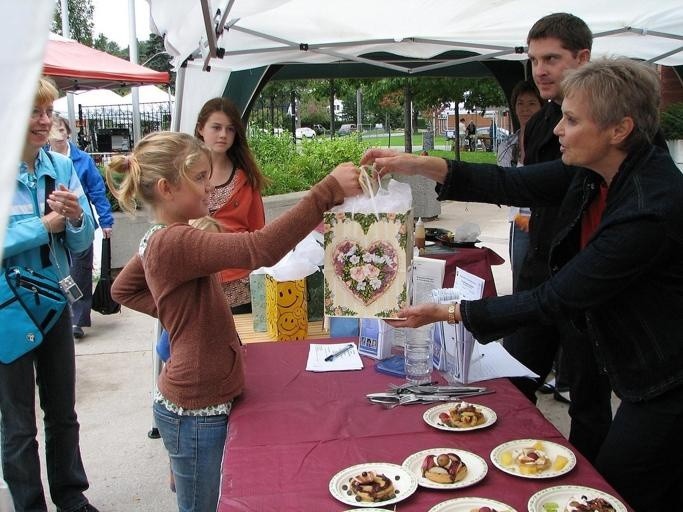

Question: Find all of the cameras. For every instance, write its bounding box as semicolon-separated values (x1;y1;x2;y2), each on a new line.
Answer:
58;275;83;305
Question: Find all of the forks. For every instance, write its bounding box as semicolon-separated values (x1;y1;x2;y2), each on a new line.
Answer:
370;381;496;404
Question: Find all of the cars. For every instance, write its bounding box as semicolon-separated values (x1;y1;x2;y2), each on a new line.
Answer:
465;127;514;149
295;128;316;140
257;128;268;135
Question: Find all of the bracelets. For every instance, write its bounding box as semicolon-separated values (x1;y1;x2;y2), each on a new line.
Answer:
447;299;458;326
68;211;83;225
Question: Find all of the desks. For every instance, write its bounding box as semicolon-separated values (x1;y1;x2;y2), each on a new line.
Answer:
214;325;633;512
421;237;506;304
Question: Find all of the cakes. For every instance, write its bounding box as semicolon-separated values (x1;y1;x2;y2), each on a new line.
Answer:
331;240;398;307
515;447;551;471
421;453;467;483
449;401;485;428
348;471;394;502
564;493;616;512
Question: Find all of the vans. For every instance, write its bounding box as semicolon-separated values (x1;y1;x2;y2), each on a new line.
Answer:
337;124;356;135
272;128;283;138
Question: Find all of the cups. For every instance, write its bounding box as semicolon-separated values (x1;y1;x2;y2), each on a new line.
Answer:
404;326;435;384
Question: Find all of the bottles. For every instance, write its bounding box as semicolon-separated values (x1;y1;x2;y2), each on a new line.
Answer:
412;216;426;249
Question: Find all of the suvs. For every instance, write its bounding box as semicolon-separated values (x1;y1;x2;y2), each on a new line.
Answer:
313;124;324;133
374;123;382;130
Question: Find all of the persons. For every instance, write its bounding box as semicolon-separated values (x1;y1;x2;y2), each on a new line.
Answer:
459;117;465;151
502;10;672;467
0;78;99;511
361;56;679;510
465;119;476;153
488;115;496;150
104;131;365;511
40;116;114;337
146;98;268;439
497;79;546;295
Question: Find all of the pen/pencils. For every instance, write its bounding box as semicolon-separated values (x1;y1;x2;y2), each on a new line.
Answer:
325;344;352;362
470;353;485;364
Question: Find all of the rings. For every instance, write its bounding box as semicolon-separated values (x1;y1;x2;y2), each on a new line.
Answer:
61;209;66;215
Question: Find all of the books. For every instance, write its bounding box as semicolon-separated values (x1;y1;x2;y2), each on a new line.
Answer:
304;341;364;374
464;341;539;386
357;252;486;385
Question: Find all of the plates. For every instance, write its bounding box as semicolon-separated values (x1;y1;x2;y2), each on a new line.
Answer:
327;462;417;508
400;446;488;492
424;227;483;249
488;438;578;480
426;497;519;512
525;484;627;512
420;402;499;432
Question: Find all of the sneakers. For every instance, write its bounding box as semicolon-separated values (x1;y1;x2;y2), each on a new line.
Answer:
73;325;85;339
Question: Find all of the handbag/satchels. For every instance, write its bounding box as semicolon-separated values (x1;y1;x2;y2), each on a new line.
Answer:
92;236;123;315
0;265;69;365
